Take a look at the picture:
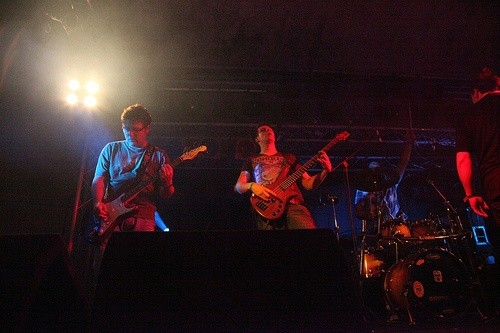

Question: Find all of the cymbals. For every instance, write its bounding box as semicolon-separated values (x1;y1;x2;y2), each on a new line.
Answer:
403;166;449;185
360;168;400;192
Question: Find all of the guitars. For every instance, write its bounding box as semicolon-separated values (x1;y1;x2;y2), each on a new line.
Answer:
92;145;207;238
250;131;350;221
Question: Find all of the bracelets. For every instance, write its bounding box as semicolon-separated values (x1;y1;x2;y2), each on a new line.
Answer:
248;181;254;190
464;192;475;202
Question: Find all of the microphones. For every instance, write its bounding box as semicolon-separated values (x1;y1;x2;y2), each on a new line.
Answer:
376;129;383;144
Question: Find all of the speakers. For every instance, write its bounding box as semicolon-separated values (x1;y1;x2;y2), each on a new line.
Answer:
91;228;371;333
0;233;91;333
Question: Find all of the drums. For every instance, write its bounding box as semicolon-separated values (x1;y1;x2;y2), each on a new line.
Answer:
369;219;412;256
408;219;439;253
383;249;472;322
351;246;389;278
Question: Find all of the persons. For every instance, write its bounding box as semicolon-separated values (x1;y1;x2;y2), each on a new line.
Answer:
90;104;176;231
454;67;500;307
357;128;416;235
233;124;332;229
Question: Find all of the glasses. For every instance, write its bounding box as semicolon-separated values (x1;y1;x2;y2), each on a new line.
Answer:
124;127;146;131
